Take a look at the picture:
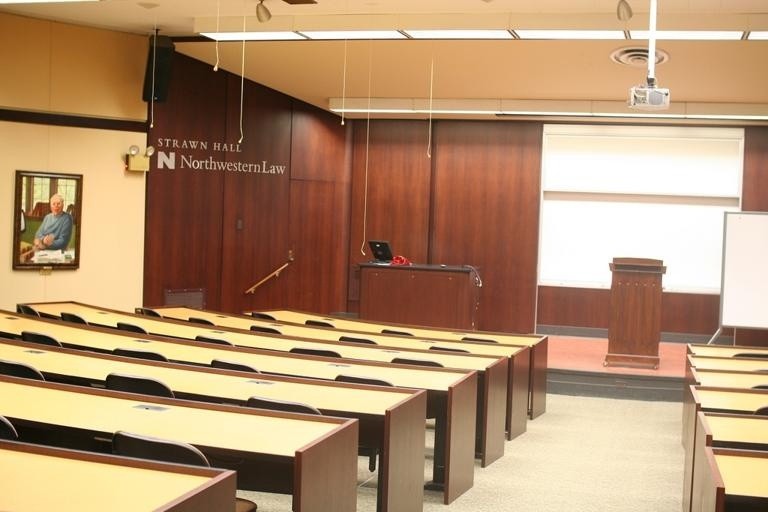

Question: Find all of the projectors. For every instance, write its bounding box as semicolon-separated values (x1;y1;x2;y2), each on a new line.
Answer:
628;87;671;110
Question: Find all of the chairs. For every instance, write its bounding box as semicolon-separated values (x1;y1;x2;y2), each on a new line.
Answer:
108;432;258;512
3;418;17;440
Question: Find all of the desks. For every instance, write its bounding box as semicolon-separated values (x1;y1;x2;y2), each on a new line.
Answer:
1;303;548;464
0;311;477;505
2;374;359;512
1;338;426;512
683;343;768;512
353;260;482;331
1;440;237;511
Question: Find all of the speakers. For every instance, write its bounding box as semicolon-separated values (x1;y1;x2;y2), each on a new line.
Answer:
142;37;176;104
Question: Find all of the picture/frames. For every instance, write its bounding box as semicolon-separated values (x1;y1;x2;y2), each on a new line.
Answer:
12;170;83;269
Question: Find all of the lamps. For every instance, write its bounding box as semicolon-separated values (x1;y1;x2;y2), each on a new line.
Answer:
126;146;154;172
255;0;272;22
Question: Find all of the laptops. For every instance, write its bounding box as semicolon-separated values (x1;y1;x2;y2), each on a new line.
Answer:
369;239;394;262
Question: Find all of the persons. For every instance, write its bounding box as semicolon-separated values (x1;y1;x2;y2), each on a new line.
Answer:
33;193;73;252
